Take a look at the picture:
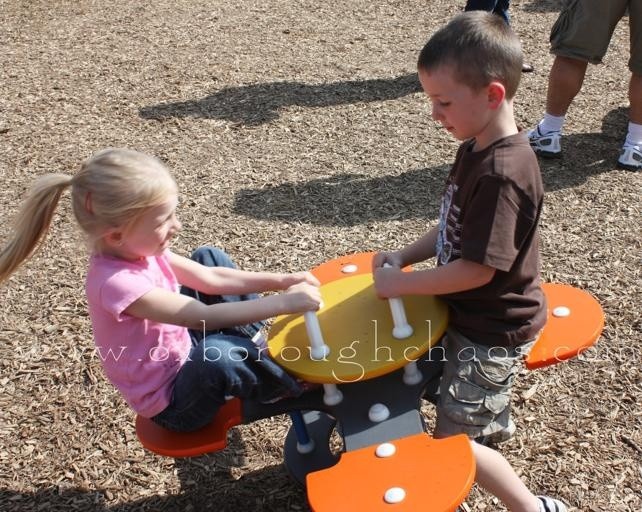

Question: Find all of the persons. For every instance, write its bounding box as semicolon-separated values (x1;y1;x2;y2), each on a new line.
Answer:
0;145;323;434
463;1;535;75
371;8;571;512
519;1;641;168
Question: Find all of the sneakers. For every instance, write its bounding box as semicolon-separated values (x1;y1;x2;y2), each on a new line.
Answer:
523;124;561;158
617;138;642;170
